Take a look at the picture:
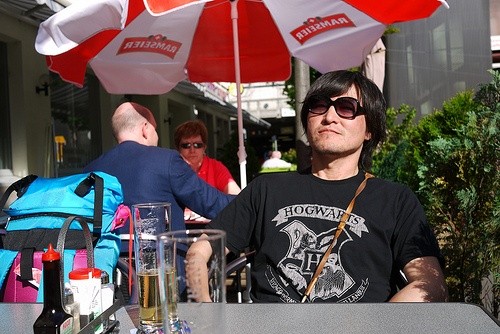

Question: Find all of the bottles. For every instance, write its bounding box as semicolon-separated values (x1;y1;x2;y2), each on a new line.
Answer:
32;243;74;334
69;267;103;334
101;270;115;320
64;288;80;334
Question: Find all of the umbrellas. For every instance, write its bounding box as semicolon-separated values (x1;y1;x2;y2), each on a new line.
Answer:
35;0;450;192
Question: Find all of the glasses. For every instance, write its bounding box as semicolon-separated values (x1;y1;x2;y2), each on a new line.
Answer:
181;143;202;149
306;93;367;120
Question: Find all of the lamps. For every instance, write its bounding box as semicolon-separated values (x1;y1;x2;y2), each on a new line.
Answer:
164;112;172;122
36;73;55;95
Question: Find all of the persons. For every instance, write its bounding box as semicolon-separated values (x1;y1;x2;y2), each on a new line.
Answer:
260;150;292;170
78;102;242;304
164;120;242;221
185;70;445;303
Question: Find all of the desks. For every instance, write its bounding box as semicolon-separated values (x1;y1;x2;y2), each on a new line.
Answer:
0;303;500;334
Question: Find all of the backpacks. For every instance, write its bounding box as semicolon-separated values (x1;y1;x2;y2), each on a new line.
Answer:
0;171;124;304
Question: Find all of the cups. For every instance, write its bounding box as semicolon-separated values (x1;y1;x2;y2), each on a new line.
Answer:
131;202;183;334
156;229;225;334
